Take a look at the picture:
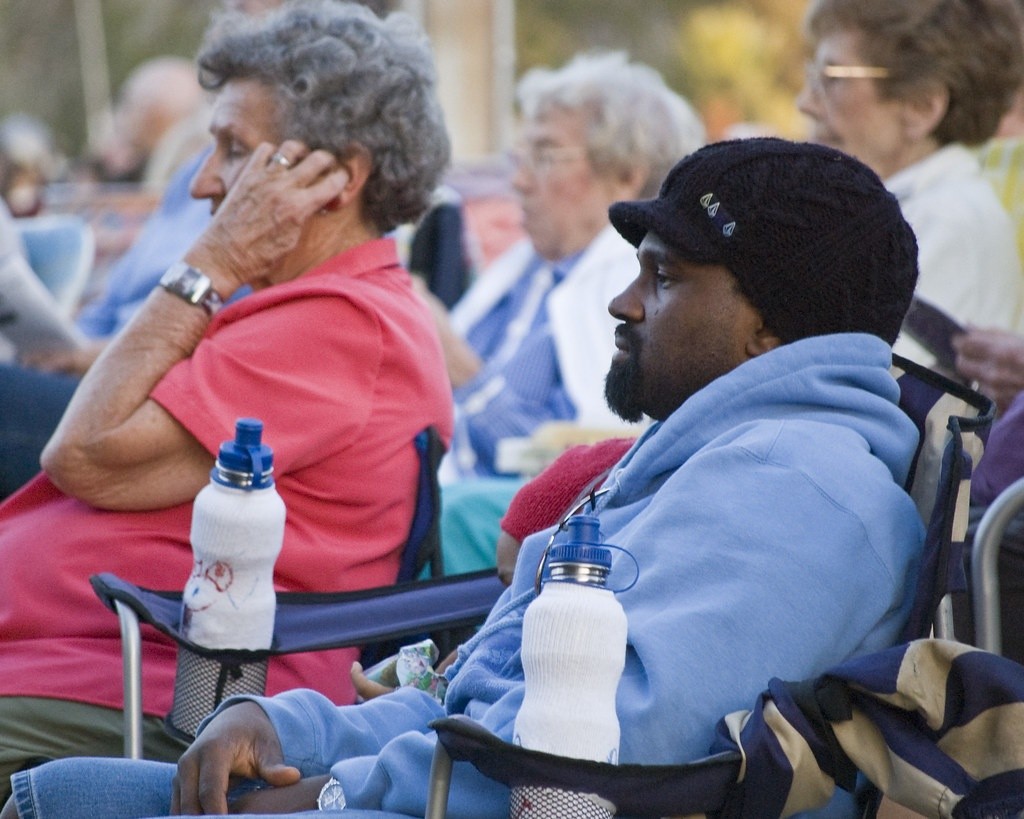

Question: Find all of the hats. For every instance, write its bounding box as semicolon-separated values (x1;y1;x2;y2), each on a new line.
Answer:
610;137;919;348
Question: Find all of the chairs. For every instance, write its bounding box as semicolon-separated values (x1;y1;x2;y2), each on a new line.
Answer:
11;187;1022;819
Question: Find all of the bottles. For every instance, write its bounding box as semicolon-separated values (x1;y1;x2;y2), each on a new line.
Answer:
170;418;286;739
512;514;640;819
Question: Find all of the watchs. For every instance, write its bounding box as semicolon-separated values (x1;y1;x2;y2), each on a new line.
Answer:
159;262;225;317
316;777;345;810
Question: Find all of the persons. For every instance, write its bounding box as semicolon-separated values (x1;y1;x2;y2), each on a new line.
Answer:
0;140;252;504
950;324;1024;417
436;53;707;486
124;55;214;183
0;137;928;819
774;0;1024;368
0;0;453;806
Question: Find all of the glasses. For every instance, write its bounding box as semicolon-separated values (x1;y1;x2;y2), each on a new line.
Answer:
803;58;905;93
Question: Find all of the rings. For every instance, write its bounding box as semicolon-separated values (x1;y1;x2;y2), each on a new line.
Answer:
273;153;290;167
